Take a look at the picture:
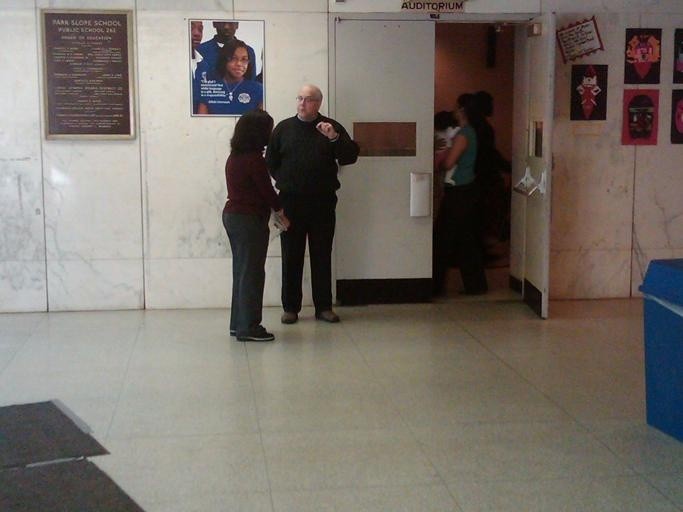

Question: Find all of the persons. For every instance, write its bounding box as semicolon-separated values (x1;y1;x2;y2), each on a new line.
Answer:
265;87;360;324
433;93;488;298
192;22;208;114
197;40;263;113
222;108;286;342
434;110;463;186
474;90;503;261
197;22;256;80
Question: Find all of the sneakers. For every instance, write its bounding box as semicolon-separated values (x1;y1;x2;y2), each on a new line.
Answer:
281;312;299;324
231;328;275;341
316;310;340;322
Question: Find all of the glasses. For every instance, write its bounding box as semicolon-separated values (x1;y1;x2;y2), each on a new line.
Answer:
296;96;321;103
232;58;248;63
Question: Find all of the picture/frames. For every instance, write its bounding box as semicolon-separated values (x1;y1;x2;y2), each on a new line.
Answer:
187;19;265;116
41;10;134;140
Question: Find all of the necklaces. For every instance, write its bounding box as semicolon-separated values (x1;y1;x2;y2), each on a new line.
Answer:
223;76;245;102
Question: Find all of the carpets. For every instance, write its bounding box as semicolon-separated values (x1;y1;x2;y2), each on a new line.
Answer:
1;456;142;511
1;401;142;467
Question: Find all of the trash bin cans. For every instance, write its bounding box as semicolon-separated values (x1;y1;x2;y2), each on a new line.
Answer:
639;258;683;442
